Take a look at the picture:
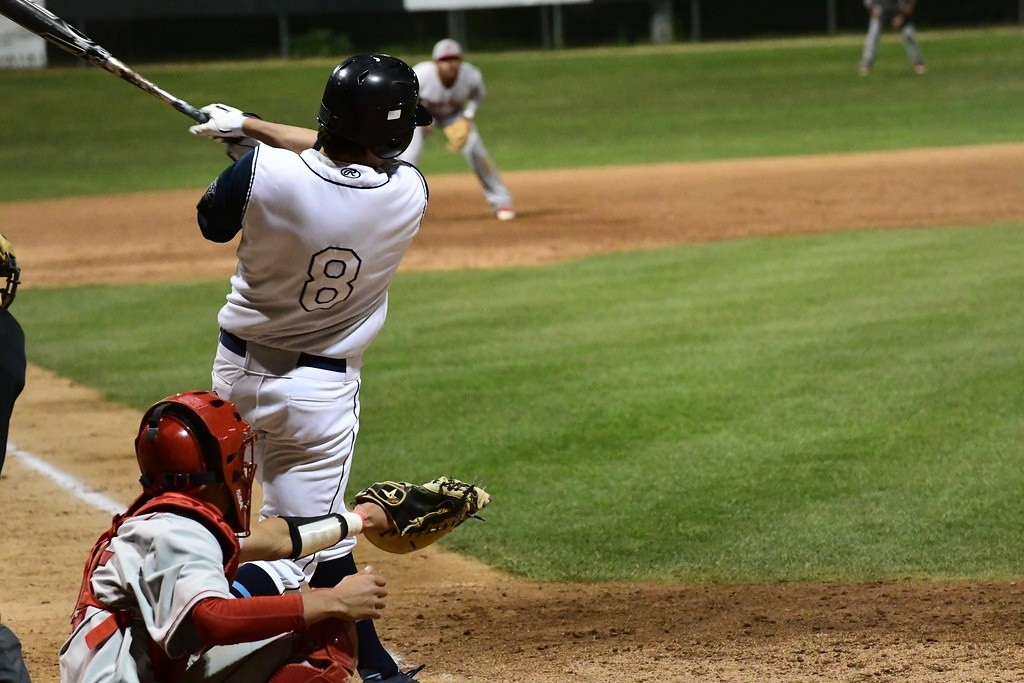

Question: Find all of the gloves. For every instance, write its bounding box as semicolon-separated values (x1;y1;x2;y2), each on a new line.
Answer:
226;137;262;163
189;102;246;140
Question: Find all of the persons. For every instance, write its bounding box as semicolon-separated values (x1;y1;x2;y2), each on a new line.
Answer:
58;390;491;683
0;234;31;683
402;40;514;220
860;0;924;74
189;55;425;683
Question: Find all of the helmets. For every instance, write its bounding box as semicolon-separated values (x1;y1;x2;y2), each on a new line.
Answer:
316;52;433;160
134;390;259;537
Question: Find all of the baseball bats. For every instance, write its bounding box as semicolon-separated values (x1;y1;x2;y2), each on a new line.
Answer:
0;0;210;125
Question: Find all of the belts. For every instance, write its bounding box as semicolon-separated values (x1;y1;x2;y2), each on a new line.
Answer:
220;329;347;374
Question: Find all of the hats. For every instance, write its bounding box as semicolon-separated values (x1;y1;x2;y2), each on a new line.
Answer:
433;40;463;62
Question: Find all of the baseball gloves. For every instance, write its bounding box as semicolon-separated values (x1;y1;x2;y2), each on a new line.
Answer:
347;474;496;557
442;117;471;154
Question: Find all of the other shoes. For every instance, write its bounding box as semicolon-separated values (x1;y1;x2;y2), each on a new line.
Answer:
496;209;515;222
361;664;425;683
